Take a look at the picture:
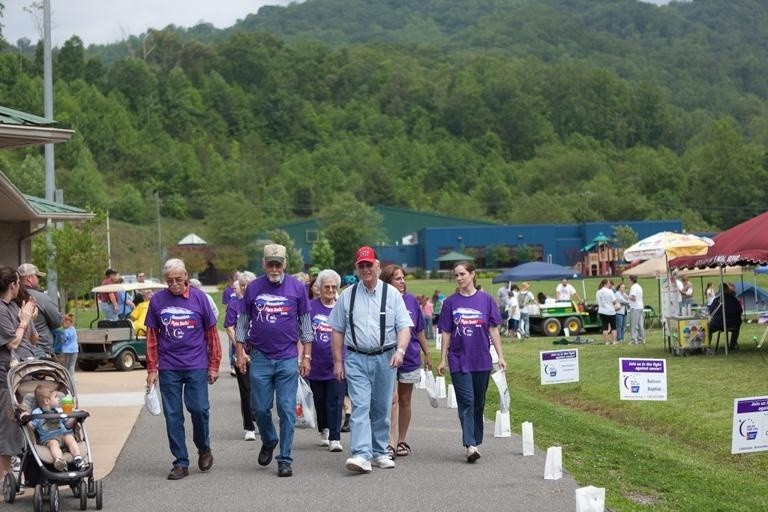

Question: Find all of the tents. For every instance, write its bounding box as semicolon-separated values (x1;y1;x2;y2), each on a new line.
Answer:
434;251;475;282
492;261;587;300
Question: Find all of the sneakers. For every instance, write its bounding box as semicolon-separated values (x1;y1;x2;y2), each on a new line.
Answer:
244;414;411;477
54;458;68;471
75;458;88;471
464;445;480;462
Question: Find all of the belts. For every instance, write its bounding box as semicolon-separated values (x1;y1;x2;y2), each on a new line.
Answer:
347;346;393;356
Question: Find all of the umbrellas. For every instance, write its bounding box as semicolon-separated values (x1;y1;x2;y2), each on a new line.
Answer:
622;212;768;276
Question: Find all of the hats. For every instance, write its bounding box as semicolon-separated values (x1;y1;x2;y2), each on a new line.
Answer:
18;263;46;277
309;267;319;276
355;246;380;265
263;243;286;266
105;269;118;275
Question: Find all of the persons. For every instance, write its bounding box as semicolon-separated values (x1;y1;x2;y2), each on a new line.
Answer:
189;279;219;319
329;246;415;473
0;265;88;495
477;281;547;341
417;290;444;340
595;276;645;345
705;282;743;349
98;269;153;336
436;261;507;462
235;244;314;477
223;271;256;440
676;277;694;305
144;259;222;479
293;267;350;451
380;265;433;459
556;278;582;304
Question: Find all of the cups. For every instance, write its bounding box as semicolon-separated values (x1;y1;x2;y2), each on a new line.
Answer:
62;398;72;413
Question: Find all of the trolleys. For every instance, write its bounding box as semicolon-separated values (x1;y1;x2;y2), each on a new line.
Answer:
665;315;714;357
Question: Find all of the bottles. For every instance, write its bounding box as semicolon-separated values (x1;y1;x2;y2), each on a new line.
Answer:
682;304;692;317
682;325;705;345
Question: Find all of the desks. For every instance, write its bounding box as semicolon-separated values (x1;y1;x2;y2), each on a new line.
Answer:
627;309;651;329
691;306;709;316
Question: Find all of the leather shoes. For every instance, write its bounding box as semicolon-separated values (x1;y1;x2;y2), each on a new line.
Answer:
198;450;213;470
168;466;189;480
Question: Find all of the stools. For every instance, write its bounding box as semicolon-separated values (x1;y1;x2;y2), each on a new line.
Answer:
716;326;739;351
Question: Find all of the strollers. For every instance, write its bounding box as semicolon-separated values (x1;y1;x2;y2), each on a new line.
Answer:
3;345;103;511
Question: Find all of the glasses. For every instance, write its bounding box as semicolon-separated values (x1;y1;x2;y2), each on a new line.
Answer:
166;277;184;284
359;264;373;269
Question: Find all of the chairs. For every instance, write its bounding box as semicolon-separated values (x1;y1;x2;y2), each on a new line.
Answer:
645;305;660;329
